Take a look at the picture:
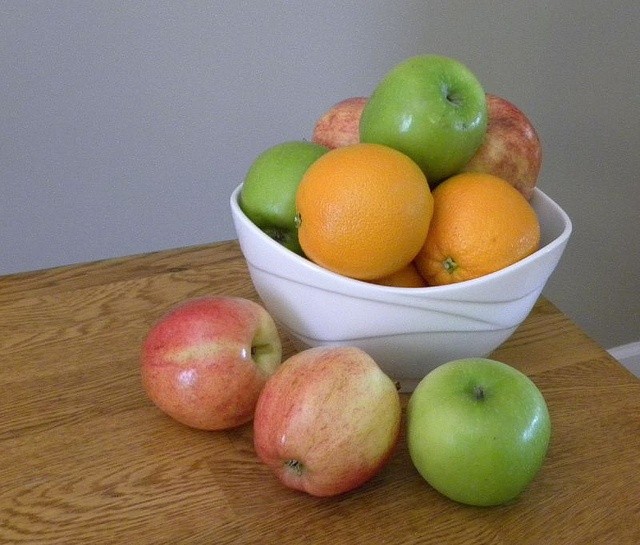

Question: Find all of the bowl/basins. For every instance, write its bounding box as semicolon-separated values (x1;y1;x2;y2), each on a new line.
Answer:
229;182;574;393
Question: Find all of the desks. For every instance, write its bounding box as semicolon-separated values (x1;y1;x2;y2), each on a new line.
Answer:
0;239;640;545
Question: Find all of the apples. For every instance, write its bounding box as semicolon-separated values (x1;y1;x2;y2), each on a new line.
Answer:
254;346;403;498
312;98;365;145
357;52;488;178
461;92;541;204
405;357;552;507
242;140;330;252
141;294;281;433
